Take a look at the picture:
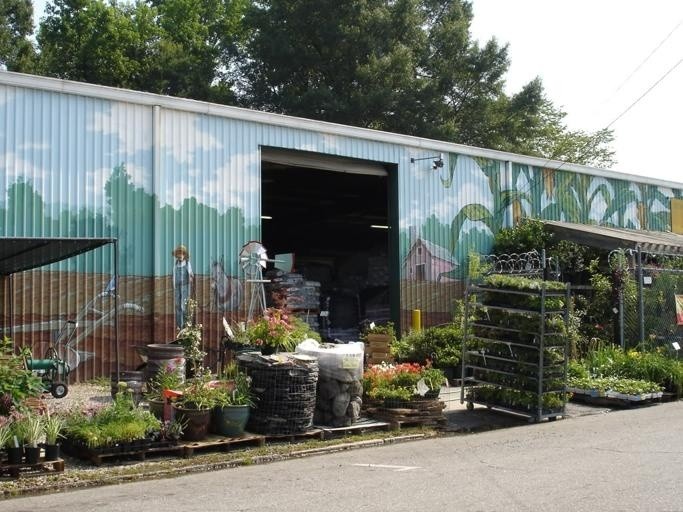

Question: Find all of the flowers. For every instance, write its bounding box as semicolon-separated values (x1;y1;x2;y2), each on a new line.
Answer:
366;359;445;389
258;303;293;345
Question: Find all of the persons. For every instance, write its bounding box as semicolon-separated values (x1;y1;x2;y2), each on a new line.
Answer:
172;246;194;331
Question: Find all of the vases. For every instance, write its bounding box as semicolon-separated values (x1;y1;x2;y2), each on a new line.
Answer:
263;340;279;355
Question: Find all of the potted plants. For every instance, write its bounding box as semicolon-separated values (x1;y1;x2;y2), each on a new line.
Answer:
457;274;567;422
1;298;257;471
367;322;395;358
568;373;664;401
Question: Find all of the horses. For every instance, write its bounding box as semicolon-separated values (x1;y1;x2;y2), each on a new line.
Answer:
208;255;243;314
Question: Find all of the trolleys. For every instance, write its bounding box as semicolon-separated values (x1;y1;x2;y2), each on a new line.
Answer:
460;279;571;424
20;292;146;398
